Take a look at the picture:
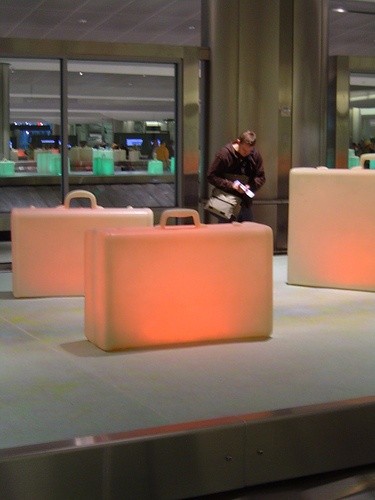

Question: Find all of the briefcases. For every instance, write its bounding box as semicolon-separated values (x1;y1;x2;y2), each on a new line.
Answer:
288;153;375;292
83;209;275;353
12;189;154;300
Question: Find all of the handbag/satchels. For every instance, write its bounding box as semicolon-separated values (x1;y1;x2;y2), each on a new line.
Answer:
202;187;243;220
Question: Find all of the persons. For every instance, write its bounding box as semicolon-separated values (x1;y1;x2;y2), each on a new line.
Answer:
128;144;147;160
206;128;266;221
154;139;172;173
